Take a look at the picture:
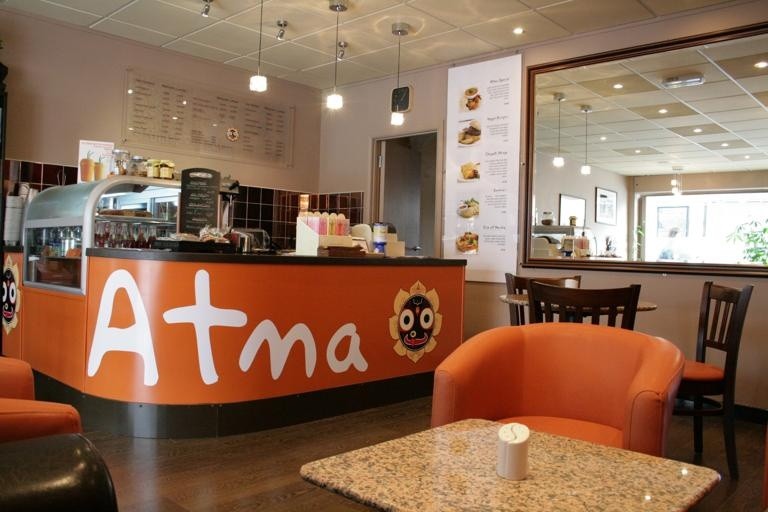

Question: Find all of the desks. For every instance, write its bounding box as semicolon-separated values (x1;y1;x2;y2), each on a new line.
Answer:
299;417;721;512
499;294;657;322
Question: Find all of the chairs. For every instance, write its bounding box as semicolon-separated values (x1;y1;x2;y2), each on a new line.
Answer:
505;273;582;325
678;281;755;482
0;355;82;441
430;321;685;457
525;277;641;330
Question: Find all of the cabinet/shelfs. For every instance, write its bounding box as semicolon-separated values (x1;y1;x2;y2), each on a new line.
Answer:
21;175;181;295
534;225;589;237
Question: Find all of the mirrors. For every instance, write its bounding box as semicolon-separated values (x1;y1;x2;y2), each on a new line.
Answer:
522;19;768;277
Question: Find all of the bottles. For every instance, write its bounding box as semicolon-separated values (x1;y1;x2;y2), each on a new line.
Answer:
111;149;174;178
93;223;101;246
127;224;136;247
102;222;112;247
146;227;157;247
113;223;125;248
137;224;146;249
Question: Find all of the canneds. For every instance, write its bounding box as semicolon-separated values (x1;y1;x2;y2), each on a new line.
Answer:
111;149;130;176
159;160;174;178
131;155;146;176
145;159;159;178
569;216;577;227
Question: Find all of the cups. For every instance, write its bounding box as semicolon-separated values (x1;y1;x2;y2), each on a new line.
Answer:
80;159;105;183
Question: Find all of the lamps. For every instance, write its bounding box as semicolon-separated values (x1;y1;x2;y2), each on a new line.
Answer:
552;93;567;168
579;104;592;175
248;0;268;92
275;19;288;39
337;41;348;59
201;0;214;17
325;0;350;110
391;22;409;126
